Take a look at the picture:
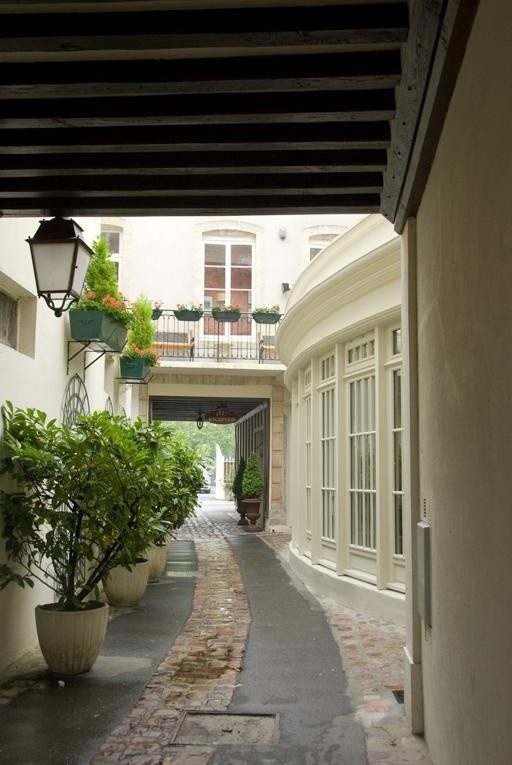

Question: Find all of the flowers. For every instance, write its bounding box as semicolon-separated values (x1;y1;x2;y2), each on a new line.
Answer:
170;299;204;311
211;302;243;312
69;290;130;324
148;295;165;309
125;342;161;366
254;304;282;312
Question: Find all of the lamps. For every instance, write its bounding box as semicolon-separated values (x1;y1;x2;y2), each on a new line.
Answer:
277;225;288;240
196;412;204;430
26;215;99;317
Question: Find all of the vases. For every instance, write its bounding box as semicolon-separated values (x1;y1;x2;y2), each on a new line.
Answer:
69;309;127;353
252;313;283;324
118;356;150;383
174;312;203;321
152;309;164;320
212;312;243;322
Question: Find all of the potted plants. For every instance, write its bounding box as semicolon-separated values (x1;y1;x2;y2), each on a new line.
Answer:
241;452;263;534
145;404;183;588
4;401;121;686
82;408;161;610
234;457;249;526
